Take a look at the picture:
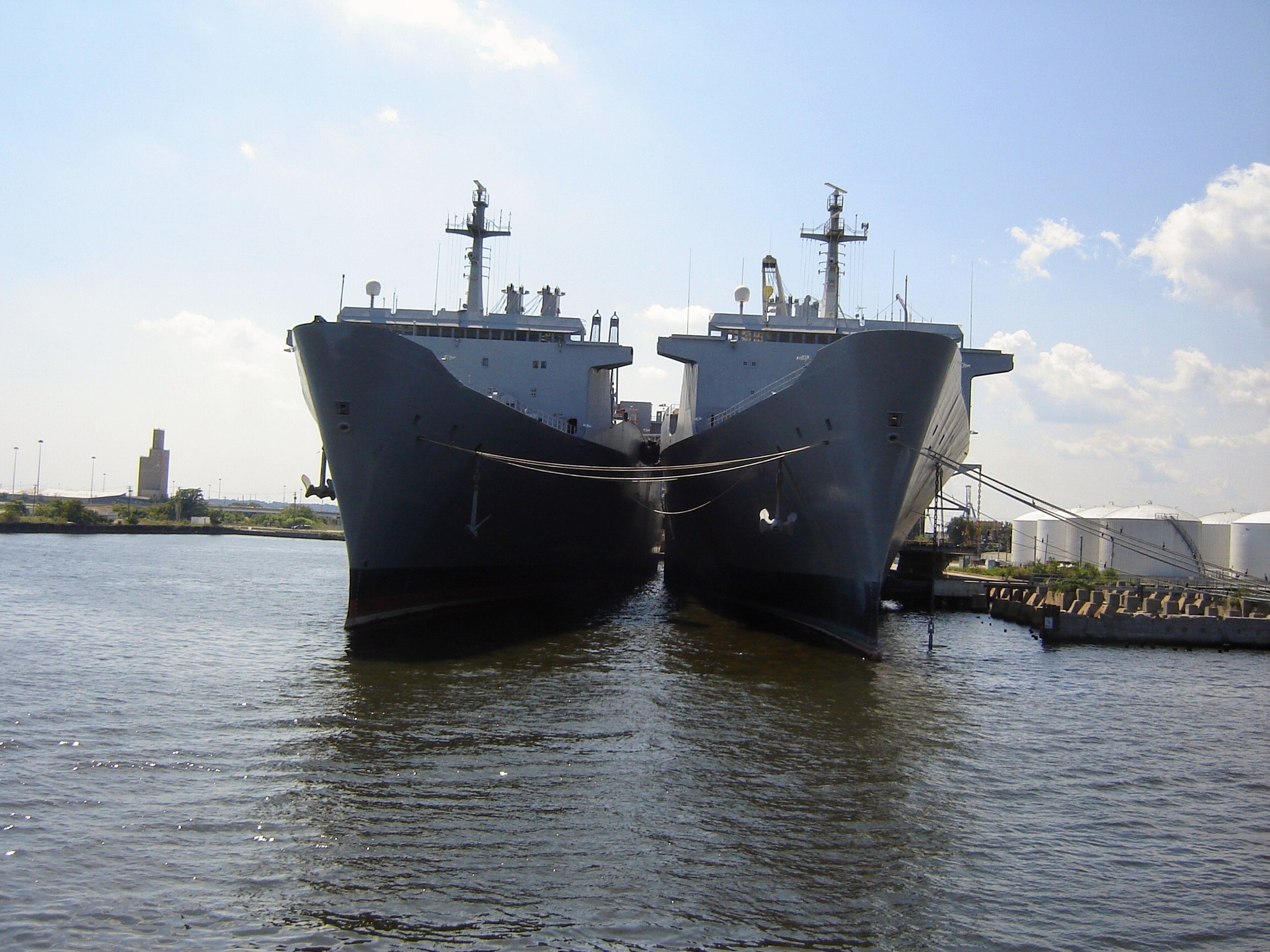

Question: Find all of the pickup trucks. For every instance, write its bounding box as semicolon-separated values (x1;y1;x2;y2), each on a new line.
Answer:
972;559;986;565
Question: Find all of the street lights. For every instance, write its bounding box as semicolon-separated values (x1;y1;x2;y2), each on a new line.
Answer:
103;474;106;492
36;440;44;502
13;447;19;500
32;485;37;513
172;481;175;498
219;478;222;507
91;457;96;506
284;486;285;510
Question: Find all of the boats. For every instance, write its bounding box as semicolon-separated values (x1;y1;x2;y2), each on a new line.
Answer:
284;175;662;635
657;182;1014;661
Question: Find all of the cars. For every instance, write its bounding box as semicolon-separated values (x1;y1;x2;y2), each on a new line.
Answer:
985;560;1010;566
